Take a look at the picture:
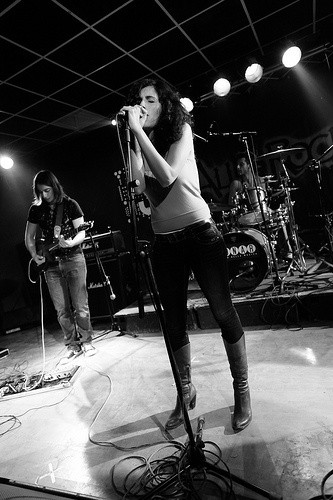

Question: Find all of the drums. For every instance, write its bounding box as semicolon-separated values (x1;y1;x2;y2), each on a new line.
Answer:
259;209;289;231
223;227;274;292
231;186;272;225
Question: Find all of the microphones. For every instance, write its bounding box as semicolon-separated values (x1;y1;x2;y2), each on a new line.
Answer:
117;109;144;118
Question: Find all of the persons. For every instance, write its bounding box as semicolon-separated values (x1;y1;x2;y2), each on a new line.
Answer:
120;79;251;433
26;169;97;365
228;152;267;206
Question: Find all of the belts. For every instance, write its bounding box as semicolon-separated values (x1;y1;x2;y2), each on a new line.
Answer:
155;219;213;243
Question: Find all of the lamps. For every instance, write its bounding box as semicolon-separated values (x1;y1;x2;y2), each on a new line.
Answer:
207;69;233;98
179;90;197;113
276;38;303;69
238;55;265;84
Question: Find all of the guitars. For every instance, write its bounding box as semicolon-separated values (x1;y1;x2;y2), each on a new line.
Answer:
32;221;94;273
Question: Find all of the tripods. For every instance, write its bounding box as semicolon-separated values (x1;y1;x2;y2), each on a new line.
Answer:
118;121;282;499
210;132;333;298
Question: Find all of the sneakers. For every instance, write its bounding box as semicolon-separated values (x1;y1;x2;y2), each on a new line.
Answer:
60;350;82;365
81;343;96;357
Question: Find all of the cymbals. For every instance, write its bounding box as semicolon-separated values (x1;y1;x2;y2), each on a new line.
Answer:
207;203;238;211
256;147;305;160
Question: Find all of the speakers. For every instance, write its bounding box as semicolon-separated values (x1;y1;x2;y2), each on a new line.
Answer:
88;257;133;321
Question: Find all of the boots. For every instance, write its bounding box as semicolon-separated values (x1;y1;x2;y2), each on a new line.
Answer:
166;343;196;429
222;332;252;433
